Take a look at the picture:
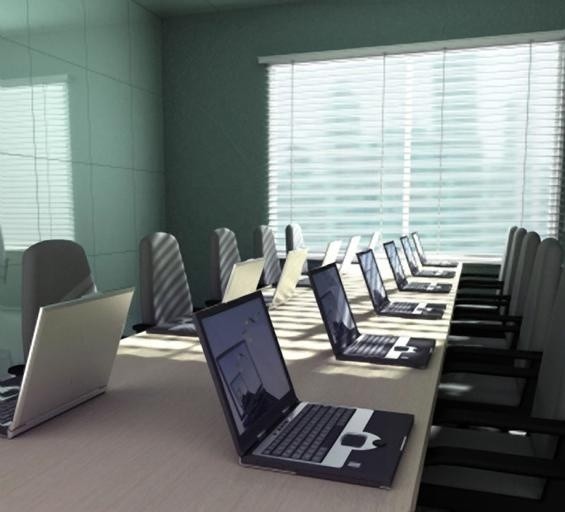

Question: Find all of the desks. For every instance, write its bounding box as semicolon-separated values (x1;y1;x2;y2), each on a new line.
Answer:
0;260;463;512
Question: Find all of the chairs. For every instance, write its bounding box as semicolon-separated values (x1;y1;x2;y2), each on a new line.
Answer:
253;225;282;287
132;232;204;333
416;226;565;512
8;239;98;377
285;223;308;274
205;227;241;307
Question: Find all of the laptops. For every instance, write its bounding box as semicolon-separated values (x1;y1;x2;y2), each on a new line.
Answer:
263;249;309;308
355;249;447;319
383;241;453;293
146;257;265;337
296;231;382;287
401;235;456;278
307;262;435;369
192;289;415;490
0;286;135;440
412;232;458;267
